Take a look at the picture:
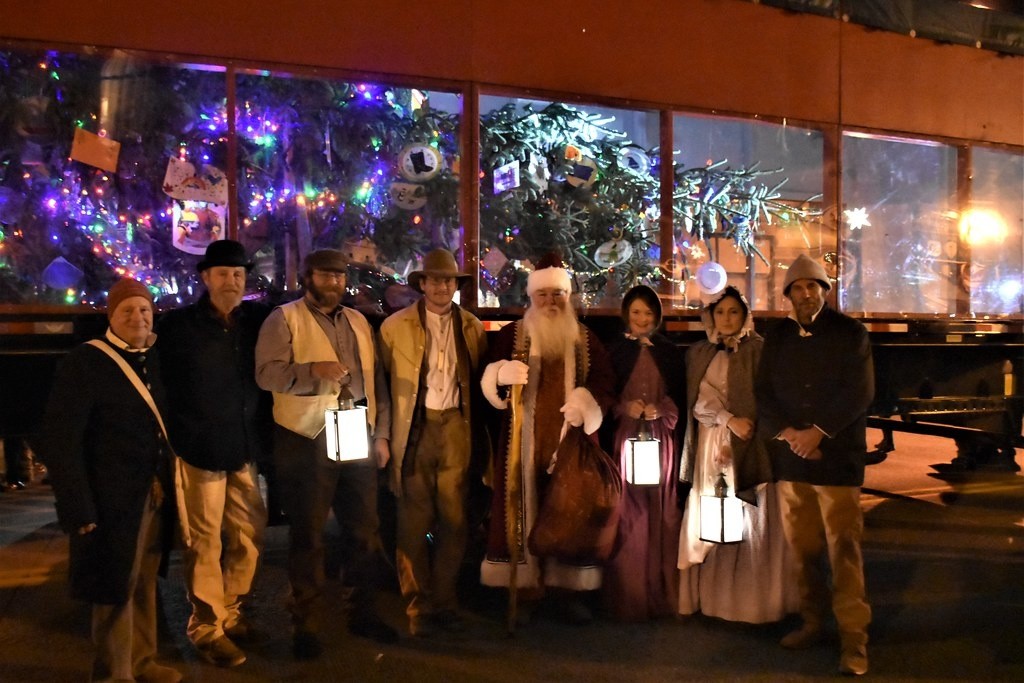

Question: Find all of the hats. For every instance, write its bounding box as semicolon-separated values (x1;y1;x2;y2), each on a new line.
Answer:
701;286;753;350
304;249;348;274
526;252;572;298
106;277;153;329
621;283;664;337
783;254;833;301
196;239;256;275
407;249;472;290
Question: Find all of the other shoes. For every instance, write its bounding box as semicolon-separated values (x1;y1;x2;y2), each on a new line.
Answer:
134;662;183;683
193;633;248;668
430;600;465;632
292;615;321;659
839;648;868;676
781;618;829;647
223;617;271;642
544;595;591;623
347;612;398;644
409;613;434;637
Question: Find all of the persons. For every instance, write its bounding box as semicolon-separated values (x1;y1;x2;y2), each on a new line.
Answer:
751;252;872;676
677;285;804;623
255;249;401;659
152;239;273;667
39;278;183;683
477;252;617;624
600;285;686;623
379;246;490;637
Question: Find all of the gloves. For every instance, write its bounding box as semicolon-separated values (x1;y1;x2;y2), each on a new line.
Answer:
498;360;529;387
560;403;584;426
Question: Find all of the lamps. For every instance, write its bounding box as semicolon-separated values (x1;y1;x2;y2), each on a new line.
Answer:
324;370;375;463
621;409;662;486
697;461;746;544
